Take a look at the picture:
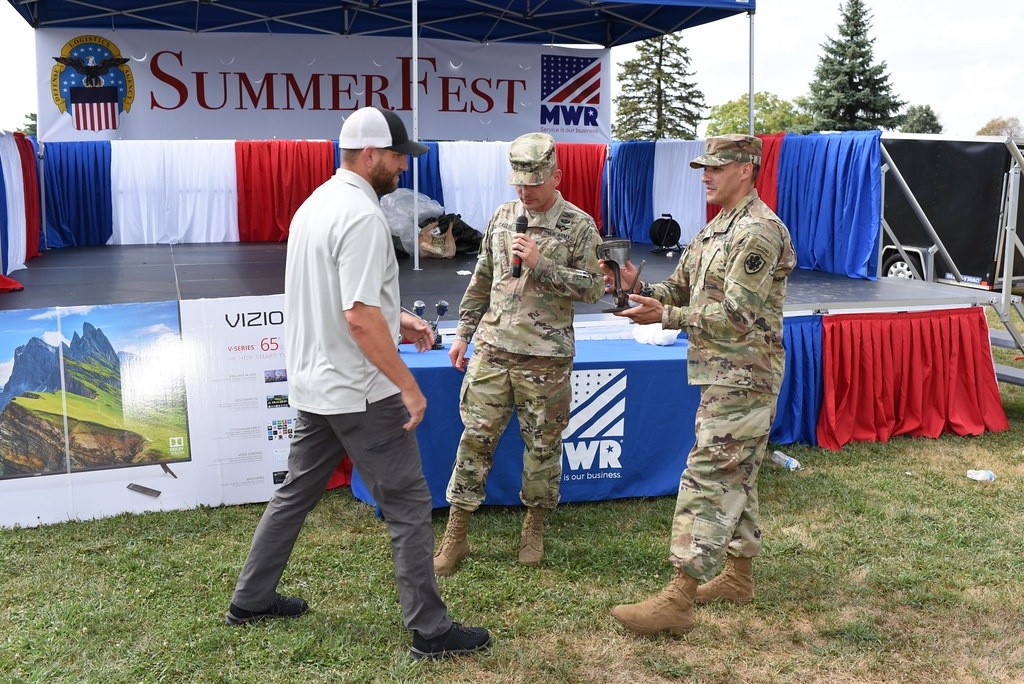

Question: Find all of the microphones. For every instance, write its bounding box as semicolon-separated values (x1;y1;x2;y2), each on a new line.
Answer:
511;216;528;278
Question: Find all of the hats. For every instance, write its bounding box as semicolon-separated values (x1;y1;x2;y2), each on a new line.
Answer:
689;133;762;170
507;131;557;185
339;106;431;155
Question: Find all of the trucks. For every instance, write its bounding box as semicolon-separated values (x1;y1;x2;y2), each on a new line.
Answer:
878;133;1024;290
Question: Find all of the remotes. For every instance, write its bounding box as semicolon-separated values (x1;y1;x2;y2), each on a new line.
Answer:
127;483;161;498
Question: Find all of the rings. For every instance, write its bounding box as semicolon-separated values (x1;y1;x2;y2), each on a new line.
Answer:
521;246;524;251
419;338;423;342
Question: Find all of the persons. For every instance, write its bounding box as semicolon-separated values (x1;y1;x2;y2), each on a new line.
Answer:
435;133;608;577
599;133;798;632
225;107;489;654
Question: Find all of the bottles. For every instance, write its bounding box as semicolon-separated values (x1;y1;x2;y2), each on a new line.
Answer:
772;451;804;472
967;470;997;481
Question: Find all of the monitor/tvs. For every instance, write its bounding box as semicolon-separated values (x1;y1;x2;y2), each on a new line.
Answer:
0;299;192;478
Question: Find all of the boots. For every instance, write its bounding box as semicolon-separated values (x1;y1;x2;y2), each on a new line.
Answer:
433;506;470;575
613;569;700;634
519;506;548;565
695;554;755;603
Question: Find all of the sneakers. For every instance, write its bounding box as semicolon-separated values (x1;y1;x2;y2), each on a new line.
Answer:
225;592;308;627
410;622;489;660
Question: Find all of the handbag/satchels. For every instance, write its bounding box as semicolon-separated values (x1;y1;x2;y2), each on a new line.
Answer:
418;223;456;259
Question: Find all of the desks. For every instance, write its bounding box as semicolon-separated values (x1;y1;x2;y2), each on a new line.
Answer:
351;338;702;519
